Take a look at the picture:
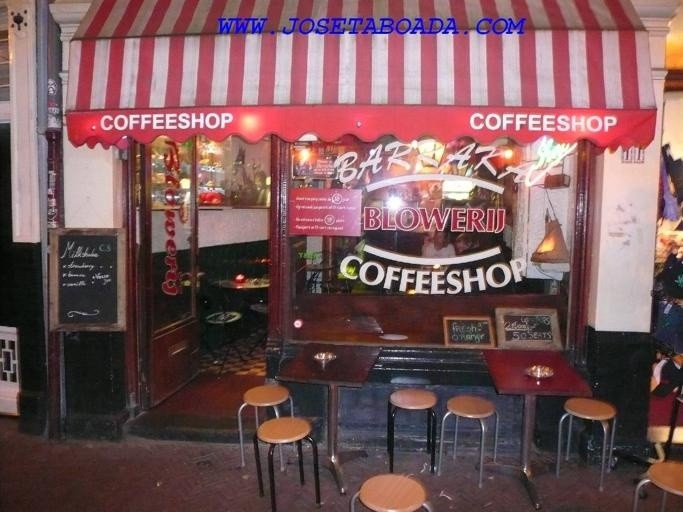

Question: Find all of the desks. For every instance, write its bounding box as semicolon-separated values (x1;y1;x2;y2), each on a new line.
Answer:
476;349;593;509
304;264;337;294
276;343;383;496
211;279;270;288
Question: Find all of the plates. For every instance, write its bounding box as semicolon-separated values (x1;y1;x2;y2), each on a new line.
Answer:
312;352;338;366
522;365;556;382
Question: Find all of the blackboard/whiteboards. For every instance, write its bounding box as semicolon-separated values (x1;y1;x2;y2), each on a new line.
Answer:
48;227;127;331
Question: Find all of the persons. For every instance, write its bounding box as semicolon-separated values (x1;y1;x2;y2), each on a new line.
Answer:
420;229;514;294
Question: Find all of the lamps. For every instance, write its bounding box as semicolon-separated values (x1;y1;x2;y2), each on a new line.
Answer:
530;189;569;263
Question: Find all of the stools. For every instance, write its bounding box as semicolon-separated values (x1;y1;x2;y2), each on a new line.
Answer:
633;461;683;510
253;418;320;512
206;312;246;378
246;302;267;356
238;385;294;472
350;474;432;512
437;396;500;487
387;389;438;473
556;397;617;490
